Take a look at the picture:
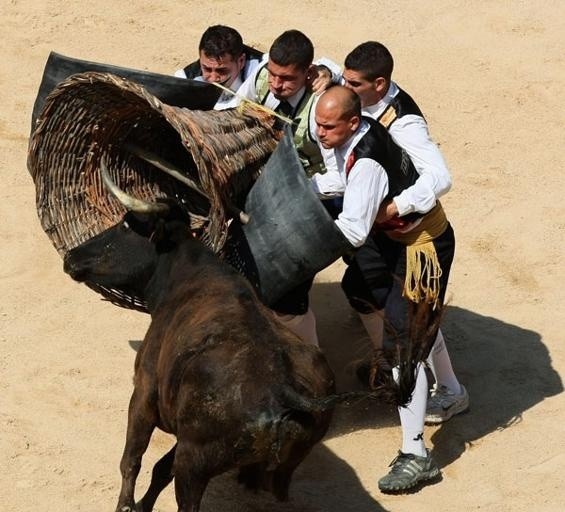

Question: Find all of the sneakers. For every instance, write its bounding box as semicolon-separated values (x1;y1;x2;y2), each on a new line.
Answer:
424;382;470;426
377;446;441;495
374;348;394;376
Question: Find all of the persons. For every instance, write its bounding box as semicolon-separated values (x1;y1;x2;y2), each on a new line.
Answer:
173;23;266;102
312;40;472;426
315;85;472;493
228;29;345;347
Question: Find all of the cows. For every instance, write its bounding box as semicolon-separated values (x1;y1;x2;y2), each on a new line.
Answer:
63;155;453;512
